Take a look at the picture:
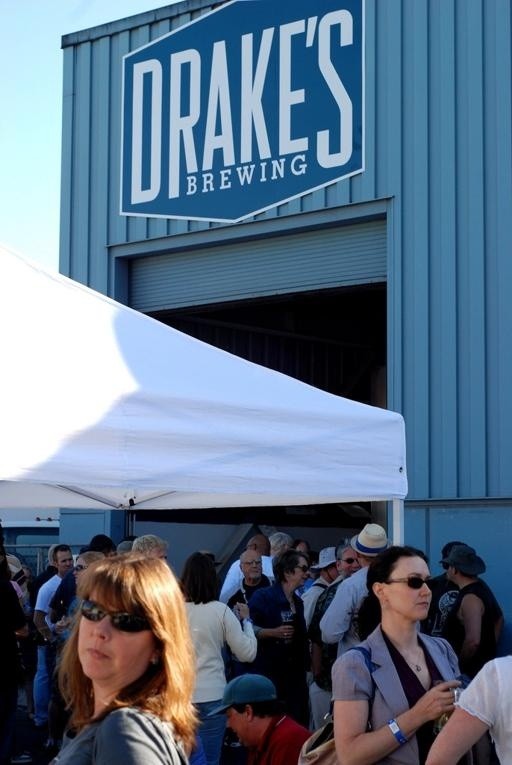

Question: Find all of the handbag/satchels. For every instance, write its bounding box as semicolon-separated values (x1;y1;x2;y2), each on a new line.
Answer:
298;721;372;765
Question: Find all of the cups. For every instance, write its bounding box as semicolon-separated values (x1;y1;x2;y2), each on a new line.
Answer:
434;681;461;736
282;620;294;645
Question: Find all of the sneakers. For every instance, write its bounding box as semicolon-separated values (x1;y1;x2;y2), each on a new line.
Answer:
11;751;32;763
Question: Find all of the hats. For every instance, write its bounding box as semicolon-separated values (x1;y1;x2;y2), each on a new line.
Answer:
350;523;389;557
201;551;222;566
207;671;278;716
310;546;336;572
440;545;487;574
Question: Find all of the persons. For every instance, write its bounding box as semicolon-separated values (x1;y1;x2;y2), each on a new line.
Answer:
1;522;511;765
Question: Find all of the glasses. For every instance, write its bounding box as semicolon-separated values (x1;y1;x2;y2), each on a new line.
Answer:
390;576;431;589
81;599;150;632
75;564;88;570
296;564;308;571
342;558;355;564
242;560;261;565
